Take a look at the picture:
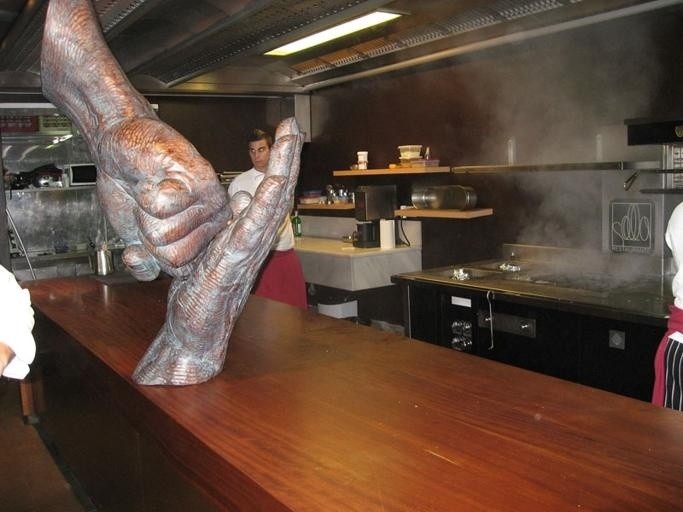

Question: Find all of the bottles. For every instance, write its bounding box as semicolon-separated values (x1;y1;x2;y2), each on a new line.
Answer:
291;211;301;237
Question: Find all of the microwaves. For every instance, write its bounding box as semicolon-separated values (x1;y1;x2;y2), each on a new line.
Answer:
69;164;96;187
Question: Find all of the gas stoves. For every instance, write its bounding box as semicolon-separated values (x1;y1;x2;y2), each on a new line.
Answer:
517;264;651;300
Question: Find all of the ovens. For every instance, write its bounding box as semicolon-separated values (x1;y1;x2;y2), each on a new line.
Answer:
440;286;582;383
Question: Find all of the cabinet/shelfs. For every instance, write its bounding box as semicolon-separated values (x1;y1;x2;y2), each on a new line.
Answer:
294;163;495;221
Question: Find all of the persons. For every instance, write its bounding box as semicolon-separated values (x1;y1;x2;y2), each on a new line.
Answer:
0;265;38;380
650;202;682;412
41;1;307;387
227;127;309;312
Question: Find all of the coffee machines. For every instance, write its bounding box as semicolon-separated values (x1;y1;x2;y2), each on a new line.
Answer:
348;184;400;248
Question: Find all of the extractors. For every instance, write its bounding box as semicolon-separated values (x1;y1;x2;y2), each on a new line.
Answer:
623;116;683;145
